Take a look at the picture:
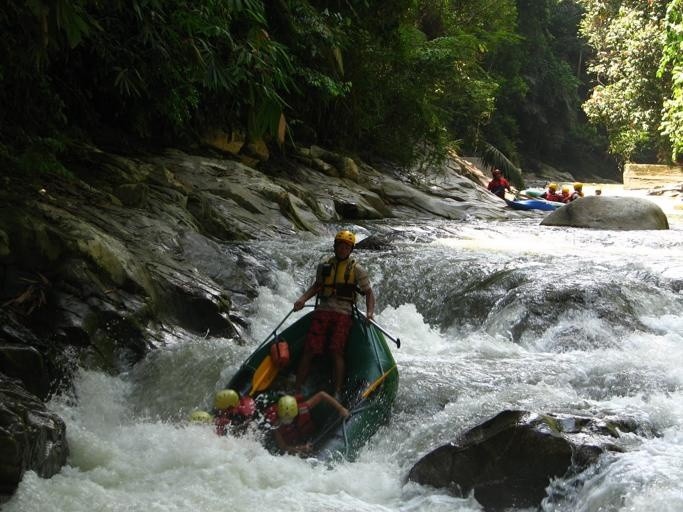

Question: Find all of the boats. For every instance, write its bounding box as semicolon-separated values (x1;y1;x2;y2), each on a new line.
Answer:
504;191;567;210
209;305;399;462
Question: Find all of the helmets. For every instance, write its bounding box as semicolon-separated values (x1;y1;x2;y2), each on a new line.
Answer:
492;168;602;193
278;396;299;421
334;230;356;244
213;390;242;409
188;411;211;429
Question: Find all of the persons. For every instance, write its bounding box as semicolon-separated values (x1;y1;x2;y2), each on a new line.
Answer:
214;389;261;421
294;231;375;400
572;181;584;198
269;390;351;455
486;168;511;202
560;188;578;203
188;410;238;437
541;182;561;202
595;186;601;196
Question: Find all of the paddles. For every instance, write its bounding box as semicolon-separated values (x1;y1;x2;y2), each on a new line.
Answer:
505;190;536;199
310;364;398;446
249;355;278;397
